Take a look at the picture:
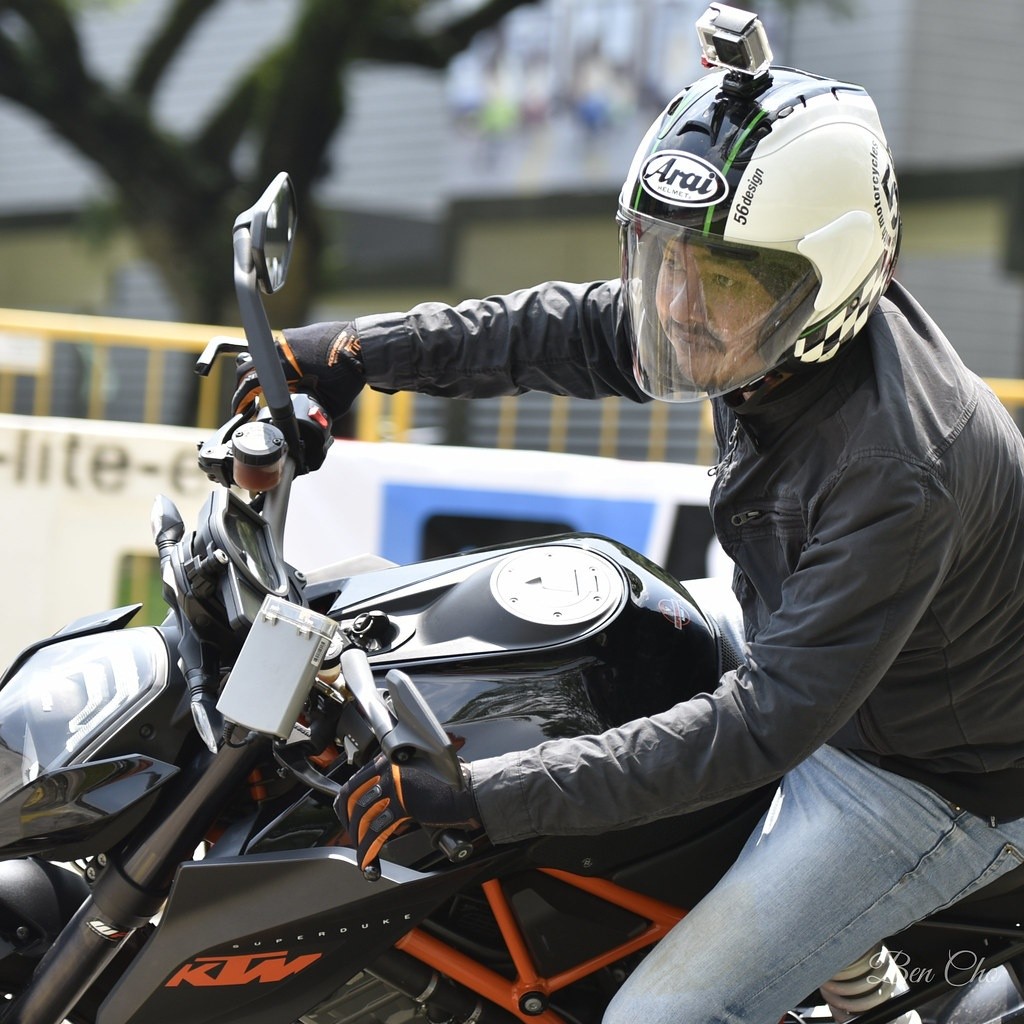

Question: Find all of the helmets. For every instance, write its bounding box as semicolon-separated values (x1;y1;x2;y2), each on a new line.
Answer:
612;65;900;403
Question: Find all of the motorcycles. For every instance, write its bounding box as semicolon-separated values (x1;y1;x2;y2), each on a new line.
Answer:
0;168;1024;1024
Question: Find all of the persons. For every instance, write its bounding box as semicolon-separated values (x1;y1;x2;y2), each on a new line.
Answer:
230;2;1023;1024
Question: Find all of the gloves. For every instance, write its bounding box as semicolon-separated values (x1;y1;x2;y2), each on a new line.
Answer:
333;749;482;873
232;320;366;423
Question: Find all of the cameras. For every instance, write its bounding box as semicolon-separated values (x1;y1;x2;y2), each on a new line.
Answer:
696;2;774;79
